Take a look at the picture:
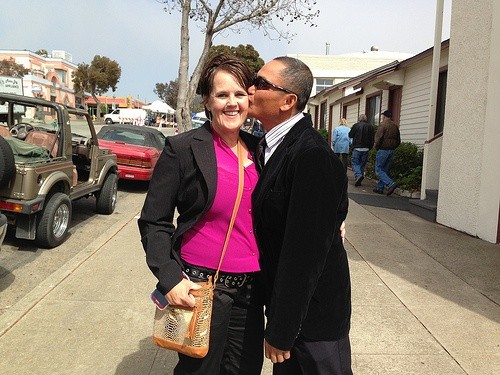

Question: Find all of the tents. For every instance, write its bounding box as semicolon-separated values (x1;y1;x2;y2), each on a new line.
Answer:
141;100;176;129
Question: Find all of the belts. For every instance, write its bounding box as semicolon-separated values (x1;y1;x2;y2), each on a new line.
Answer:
180;264;258;289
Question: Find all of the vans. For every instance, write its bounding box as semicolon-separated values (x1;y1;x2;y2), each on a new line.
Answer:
192;112;207;120
104;108;147;127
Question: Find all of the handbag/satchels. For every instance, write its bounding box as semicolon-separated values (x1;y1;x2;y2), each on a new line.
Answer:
152;275;214;358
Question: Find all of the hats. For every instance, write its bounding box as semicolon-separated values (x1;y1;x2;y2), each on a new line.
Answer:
382;111;392;118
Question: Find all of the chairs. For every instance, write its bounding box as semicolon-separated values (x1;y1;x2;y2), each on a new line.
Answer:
107;134;153;147
0;126;11;137
25;131;78;186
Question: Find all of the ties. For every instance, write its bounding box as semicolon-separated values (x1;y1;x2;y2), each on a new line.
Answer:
254;137;266;175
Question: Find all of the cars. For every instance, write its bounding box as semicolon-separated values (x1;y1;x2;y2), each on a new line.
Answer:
176;120;205;136
96;123;167;182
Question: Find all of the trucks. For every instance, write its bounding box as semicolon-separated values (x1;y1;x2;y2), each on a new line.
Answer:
0;76;26;125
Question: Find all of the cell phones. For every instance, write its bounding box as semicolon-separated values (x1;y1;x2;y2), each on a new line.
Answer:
149;271;190;311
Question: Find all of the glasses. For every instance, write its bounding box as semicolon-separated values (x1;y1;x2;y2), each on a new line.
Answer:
251;76;299;102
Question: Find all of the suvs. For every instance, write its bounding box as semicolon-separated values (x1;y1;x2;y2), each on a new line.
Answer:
0;91;120;249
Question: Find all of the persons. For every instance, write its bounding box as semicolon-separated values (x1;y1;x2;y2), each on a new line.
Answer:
250;118;265;138
330;117;354;173
242;118;252;134
372;110;402;196
246;57;353;375
348;113;375;187
138;52;271;374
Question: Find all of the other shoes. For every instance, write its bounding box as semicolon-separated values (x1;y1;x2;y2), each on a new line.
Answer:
387;183;398;195
355;174;363;187
373;189;383;193
